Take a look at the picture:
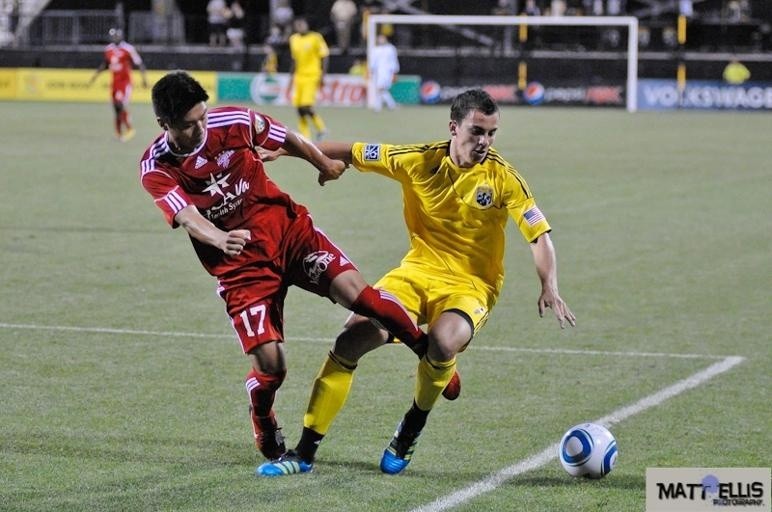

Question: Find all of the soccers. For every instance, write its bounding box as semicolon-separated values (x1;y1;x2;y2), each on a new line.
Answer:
560;423;619;480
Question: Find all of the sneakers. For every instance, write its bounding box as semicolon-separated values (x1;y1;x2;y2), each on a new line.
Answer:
256;450;315;476
381;417;420;476
249;402;286;461
418;335;461;400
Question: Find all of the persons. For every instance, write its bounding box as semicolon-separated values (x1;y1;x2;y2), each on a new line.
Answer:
285;15;331;142
204;0;397;73
254;88;575;477
488;0;771;83
366;31;400;112
139;72;462;460
87;27;149;141
